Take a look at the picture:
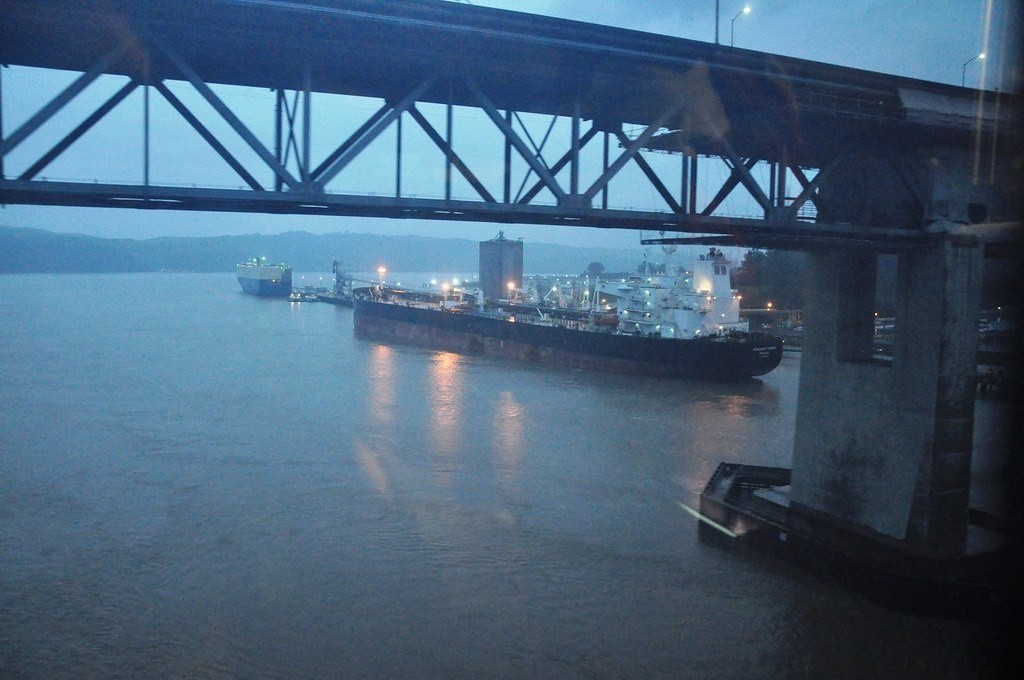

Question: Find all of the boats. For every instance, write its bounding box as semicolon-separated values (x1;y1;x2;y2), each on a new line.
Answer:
288;293;318;304
349;229;784;382
236;256;292;297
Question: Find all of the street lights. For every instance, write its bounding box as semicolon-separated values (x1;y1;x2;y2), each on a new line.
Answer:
731;7;751;47
962;53;986;87
